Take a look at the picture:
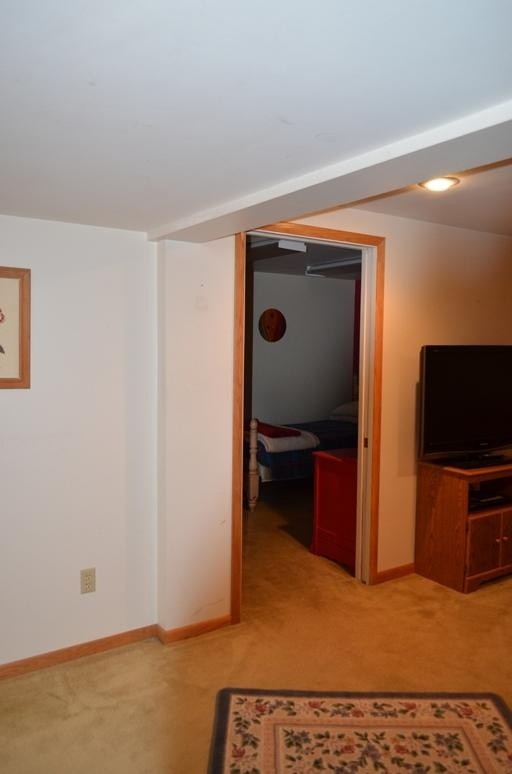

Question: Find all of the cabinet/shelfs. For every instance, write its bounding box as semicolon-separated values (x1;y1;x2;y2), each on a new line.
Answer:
414;461;512;593
310;449;358;570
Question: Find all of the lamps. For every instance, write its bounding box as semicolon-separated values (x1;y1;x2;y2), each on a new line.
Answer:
248;240;307;263
305;255;362;276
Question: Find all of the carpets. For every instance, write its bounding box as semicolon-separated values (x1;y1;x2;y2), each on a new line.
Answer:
204;688;512;774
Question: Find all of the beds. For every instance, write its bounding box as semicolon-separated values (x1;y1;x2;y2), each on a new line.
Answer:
244;411;358;511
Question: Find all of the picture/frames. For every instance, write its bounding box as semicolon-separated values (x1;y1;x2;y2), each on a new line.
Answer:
0;265;31;389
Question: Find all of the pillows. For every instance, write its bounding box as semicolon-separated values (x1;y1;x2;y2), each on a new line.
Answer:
329;400;358;423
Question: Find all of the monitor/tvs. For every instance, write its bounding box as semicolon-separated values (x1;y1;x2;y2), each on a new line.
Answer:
417;345;512;469
